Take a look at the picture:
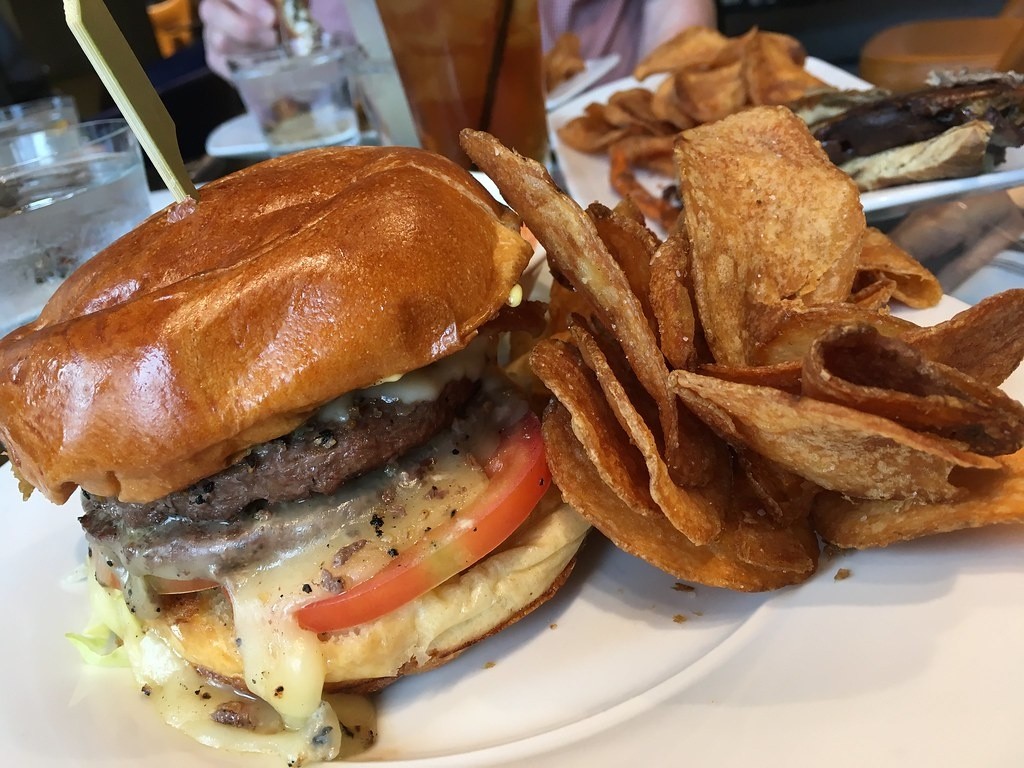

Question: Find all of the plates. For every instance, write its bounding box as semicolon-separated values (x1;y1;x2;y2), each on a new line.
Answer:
325;535;774;766
543;55;1024;228
204;107;358;157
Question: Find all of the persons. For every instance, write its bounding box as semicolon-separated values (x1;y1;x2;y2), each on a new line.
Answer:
196;0;722;176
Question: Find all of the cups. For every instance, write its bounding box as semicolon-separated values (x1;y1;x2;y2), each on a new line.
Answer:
0;93;80;167
1;118;151;338
228;41;361;156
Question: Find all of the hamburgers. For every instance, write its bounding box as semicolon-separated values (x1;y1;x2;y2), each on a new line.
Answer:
0;144;594;700
